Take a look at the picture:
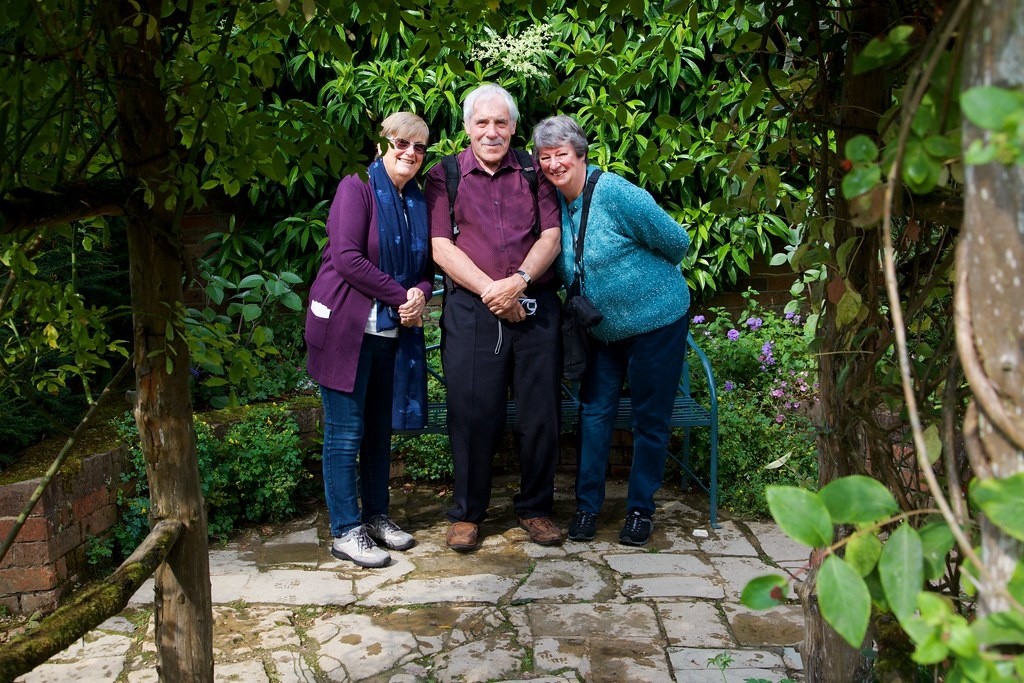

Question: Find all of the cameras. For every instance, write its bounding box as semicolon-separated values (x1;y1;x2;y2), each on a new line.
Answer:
518;298;537;315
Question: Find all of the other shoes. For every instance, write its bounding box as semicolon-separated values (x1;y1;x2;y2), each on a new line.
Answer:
518;516;563;545
446;521;478;551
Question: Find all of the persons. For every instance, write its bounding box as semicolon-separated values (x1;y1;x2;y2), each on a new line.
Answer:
428;86;563;551
532;117;692;544
302;112;435;569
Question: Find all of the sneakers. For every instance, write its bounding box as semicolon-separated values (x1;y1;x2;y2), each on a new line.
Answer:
618;511;652;544
567;509;597;540
364;514;415;550
331;525;390;567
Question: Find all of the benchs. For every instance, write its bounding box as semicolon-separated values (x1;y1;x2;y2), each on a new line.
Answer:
356;275;721;529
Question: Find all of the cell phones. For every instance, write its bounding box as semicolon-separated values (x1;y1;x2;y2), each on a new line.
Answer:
388;307;400;319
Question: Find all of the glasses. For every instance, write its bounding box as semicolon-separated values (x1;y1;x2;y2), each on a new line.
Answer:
390;138;428;154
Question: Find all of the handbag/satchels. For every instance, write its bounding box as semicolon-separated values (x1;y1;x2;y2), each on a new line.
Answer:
561;273;587;380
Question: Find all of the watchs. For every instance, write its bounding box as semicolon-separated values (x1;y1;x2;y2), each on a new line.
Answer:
516;270;531;286
